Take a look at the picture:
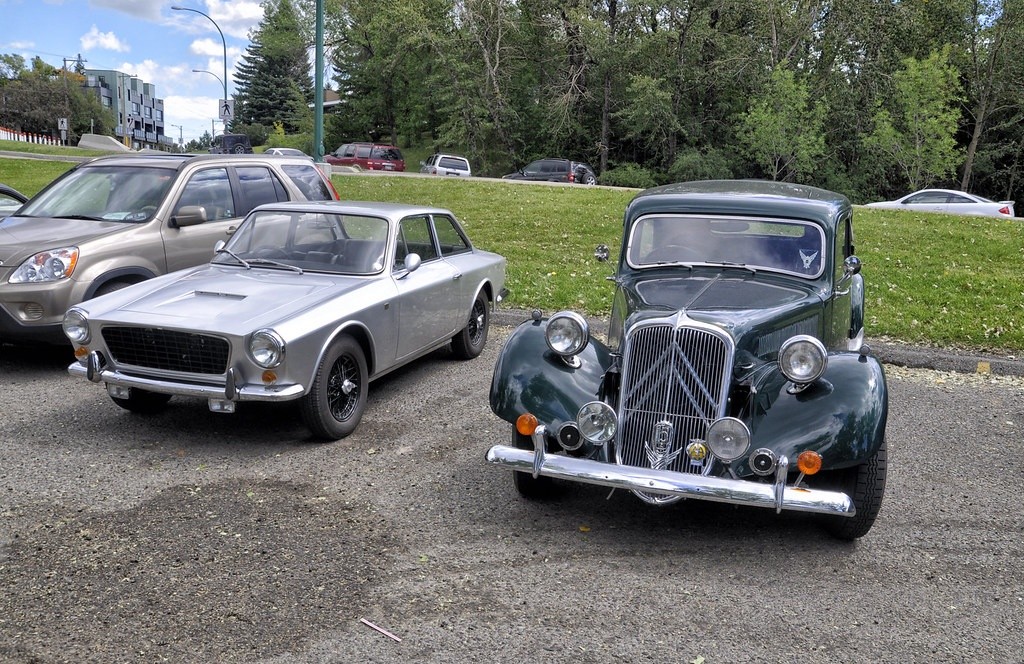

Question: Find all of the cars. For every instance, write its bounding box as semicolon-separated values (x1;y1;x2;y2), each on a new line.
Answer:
61;199;509;441
420;152;471;177
484;179;890;539
862;187;1016;220
261;148;315;161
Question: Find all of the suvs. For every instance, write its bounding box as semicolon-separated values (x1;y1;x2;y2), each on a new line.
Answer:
501;158;598;186
322;141;406;173
0;154;346;345
208;134;255;155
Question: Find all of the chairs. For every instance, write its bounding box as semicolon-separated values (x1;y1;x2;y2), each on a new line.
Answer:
193;186;223;223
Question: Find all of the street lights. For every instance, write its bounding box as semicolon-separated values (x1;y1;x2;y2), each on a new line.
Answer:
171;6;230;134
171;124;182;154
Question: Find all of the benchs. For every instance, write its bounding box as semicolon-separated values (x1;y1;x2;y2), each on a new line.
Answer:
316;239;464;264
